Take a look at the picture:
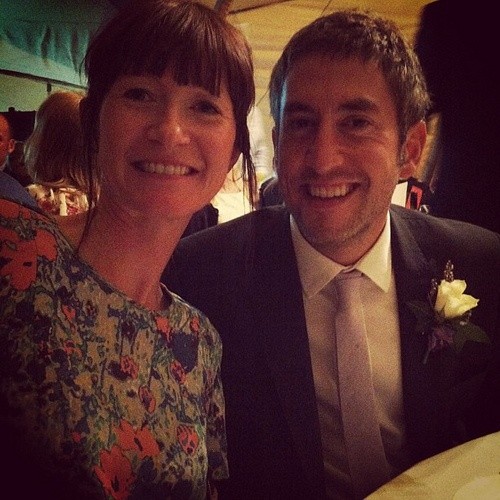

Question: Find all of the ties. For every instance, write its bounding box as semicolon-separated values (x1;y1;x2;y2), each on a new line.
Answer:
333;270;392;500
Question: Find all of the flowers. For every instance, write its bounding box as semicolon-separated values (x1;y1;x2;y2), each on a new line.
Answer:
407;260;488;366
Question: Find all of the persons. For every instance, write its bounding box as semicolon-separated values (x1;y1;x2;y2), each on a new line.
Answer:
1;90;217;241
160;10;500;500
403;0;500;233
0;0;259;500
